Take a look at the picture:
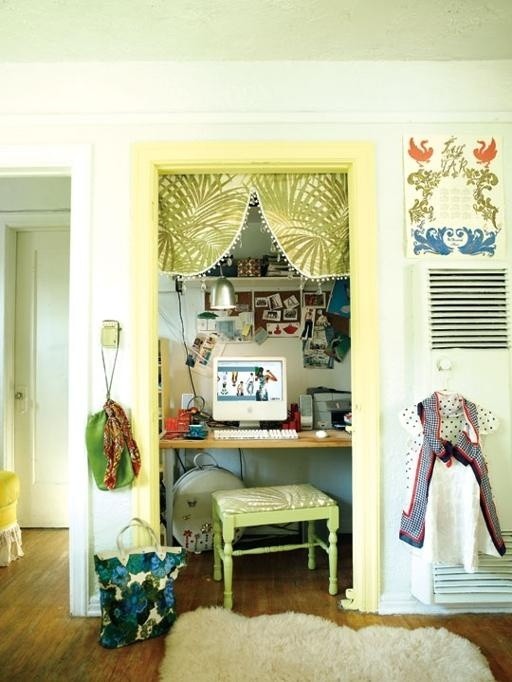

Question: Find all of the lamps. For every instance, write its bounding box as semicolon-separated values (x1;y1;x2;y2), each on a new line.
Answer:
209;263;238;311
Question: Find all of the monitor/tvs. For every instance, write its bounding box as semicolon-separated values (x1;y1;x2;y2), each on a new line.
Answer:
212;357;287;427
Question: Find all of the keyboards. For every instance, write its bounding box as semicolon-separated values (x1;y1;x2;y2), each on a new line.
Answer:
215;428;300;440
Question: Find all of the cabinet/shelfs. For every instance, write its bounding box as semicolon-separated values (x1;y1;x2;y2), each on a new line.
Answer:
158;337;170;549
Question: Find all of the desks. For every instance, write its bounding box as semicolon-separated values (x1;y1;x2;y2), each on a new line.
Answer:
159;419;351;590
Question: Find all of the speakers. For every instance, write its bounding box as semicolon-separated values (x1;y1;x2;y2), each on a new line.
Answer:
301;394;313;430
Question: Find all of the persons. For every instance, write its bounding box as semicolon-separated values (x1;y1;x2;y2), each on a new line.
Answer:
217;367;278;401
301;308;313;338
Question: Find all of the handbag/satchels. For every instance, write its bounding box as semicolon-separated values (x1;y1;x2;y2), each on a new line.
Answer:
85;408;140;491
93;518;186;649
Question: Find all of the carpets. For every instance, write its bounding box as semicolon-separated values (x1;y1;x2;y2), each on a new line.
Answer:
157;604;495;682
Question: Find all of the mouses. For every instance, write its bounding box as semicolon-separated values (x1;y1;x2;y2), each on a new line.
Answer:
317;429;330;439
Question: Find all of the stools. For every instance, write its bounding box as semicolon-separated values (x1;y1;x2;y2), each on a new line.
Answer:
209;482;340;610
0;469;27;568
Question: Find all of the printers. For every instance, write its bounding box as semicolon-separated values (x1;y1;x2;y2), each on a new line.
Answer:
314;389;352;430
